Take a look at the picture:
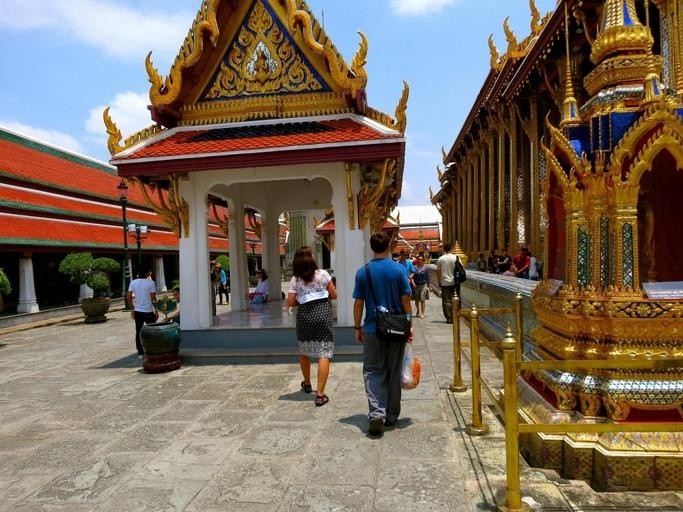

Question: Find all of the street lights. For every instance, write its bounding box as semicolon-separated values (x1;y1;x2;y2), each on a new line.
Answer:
248;239;257;254
116;176;134;311
127;222;150;265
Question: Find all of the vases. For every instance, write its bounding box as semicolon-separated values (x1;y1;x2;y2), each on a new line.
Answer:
138;321;183;372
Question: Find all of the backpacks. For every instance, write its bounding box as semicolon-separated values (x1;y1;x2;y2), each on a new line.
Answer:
454;256;467;282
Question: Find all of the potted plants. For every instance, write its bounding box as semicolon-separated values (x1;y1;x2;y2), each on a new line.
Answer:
57;251;121;326
215;255;230;292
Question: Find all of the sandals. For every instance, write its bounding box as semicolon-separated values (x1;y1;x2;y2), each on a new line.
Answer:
315;394;329;406
301;381;312;393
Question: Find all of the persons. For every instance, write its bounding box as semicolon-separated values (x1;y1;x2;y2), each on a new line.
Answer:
288;246;337;406
248;269;269;302
217;266;229;305
395;249;417;290
411;255;433;319
126;265;157;360
352;233;414;434
476;245;543;281
164;288;180;325
437;244;465;324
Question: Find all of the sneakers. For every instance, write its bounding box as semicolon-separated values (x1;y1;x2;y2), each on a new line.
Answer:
368;415;385;436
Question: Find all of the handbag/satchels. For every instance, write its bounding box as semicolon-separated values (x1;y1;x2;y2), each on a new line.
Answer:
375;306;411;342
252;295;264;304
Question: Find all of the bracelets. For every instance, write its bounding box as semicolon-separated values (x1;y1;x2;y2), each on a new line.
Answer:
353;326;361;330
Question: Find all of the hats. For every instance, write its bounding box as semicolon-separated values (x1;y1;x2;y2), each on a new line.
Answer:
521;246;528;253
215;263;222;268
